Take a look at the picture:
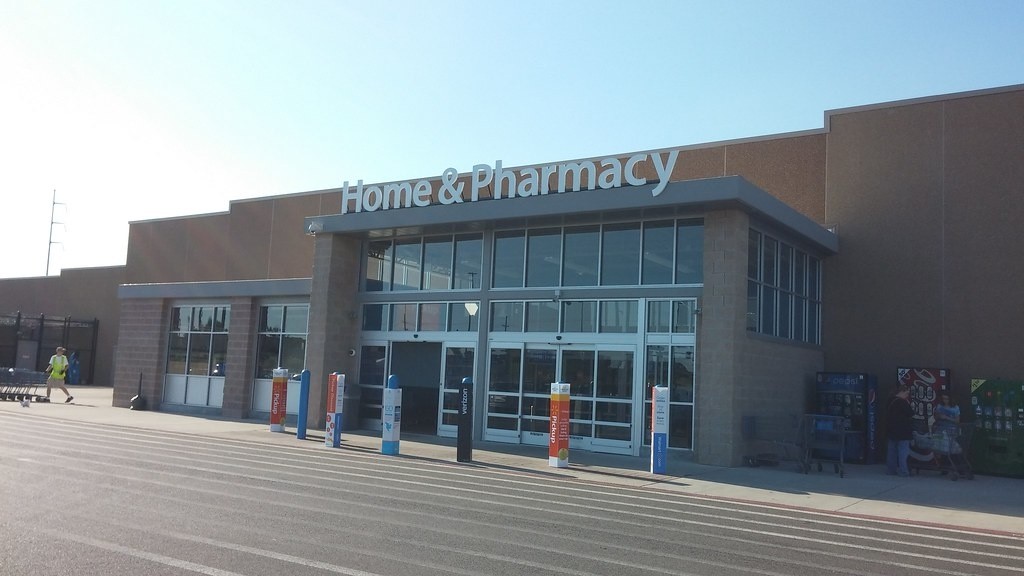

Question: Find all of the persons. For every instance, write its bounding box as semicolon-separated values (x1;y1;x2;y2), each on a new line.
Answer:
935;392;960;474
42;346;74;404
886;385;915;477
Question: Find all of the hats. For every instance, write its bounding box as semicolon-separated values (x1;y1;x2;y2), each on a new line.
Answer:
56;347;67;352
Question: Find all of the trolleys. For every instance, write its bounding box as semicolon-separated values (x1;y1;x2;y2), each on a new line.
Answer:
908;421;976;482
0;366;52;404
801;413;853;478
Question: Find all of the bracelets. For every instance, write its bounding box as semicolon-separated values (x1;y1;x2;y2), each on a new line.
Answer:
44;371;47;373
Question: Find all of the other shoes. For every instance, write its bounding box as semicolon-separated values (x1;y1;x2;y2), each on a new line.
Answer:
42;398;50;403
65;396;73;403
